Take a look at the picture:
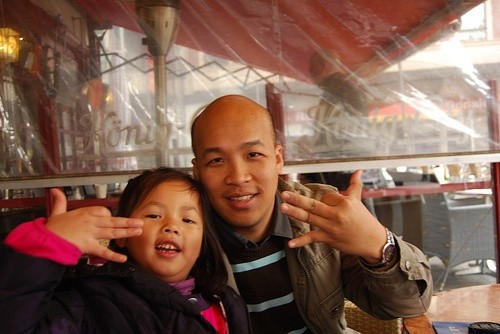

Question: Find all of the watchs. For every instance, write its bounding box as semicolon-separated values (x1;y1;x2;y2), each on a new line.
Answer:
358;225;397;269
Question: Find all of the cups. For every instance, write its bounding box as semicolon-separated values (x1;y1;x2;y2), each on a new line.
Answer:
467;322;500;334
448;164;462;176
95;184;107;198
422;166;432;173
72;186;85;199
470;163;482;176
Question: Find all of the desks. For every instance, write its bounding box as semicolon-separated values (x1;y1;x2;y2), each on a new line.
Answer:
453;188;495;277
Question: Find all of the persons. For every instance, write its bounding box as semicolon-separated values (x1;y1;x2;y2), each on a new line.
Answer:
0;164;253;333
188;94;435;334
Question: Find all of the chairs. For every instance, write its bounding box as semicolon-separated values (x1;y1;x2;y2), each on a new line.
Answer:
386;169;496;291
